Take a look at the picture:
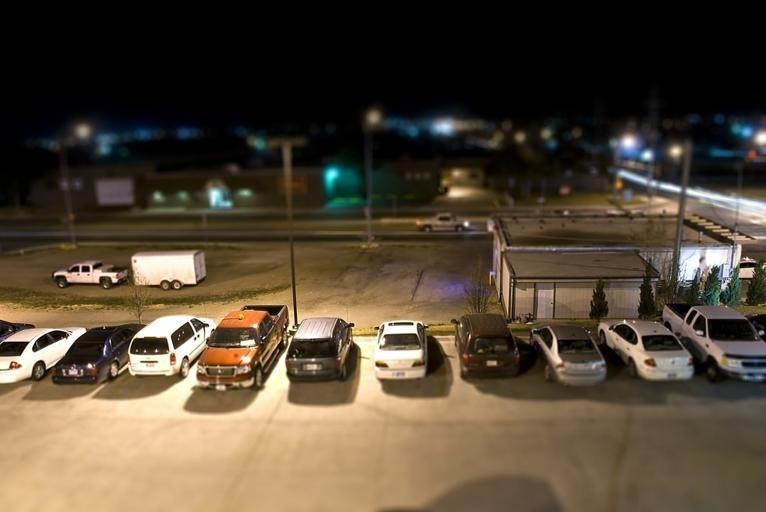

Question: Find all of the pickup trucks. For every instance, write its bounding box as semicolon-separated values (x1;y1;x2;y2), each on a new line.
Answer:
662;302;765;384
199;305;289;391
51;260;127;289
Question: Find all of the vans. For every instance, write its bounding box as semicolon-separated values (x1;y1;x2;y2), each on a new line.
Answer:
130;249;207;291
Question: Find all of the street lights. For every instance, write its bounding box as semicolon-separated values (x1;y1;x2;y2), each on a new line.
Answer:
360;109;384;246
262;133;310;324
59;118;97;245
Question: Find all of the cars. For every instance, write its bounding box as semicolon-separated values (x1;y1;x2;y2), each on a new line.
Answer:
415;211;469;231
127;314;216;379
0;326;86;384
53;324;144;385
0;321;34;345
